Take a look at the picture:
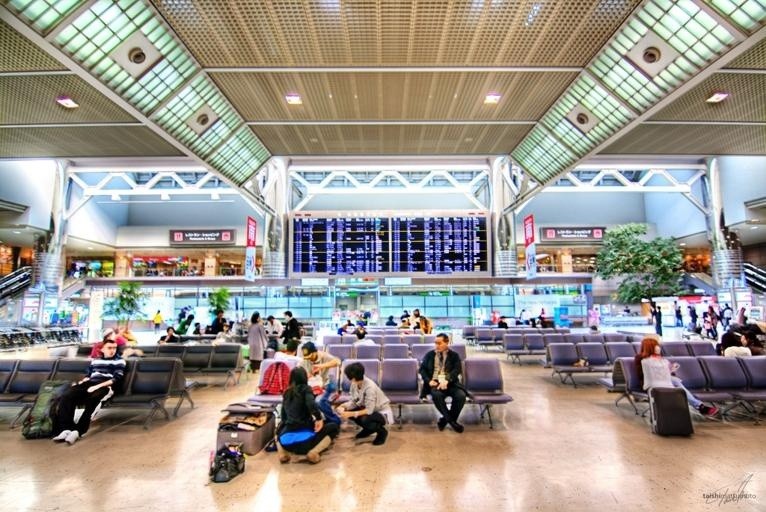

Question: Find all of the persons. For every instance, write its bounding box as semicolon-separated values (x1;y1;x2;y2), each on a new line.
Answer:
50;309;59;324
418;333;466;434
229;264;235;275
335;361;397;444
338;307;433;339
296;342;342;426
274;366;340;463
185;266;197;276
635;338;719;417
491;307;548;328
89;309;301;375
52;340;127;445
624;305;632;314
593;307;602;326
649;301;766;356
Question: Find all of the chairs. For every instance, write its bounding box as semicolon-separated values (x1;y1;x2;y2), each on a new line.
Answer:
0;334;295;427
515;328;766;423
296;327;514;434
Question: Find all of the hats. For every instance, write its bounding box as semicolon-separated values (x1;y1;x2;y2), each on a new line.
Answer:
302;342;313;359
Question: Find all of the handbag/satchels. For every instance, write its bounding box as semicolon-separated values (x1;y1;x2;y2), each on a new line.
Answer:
215;442;245;482
22;380;72;439
307;367;324;388
287;339;300;351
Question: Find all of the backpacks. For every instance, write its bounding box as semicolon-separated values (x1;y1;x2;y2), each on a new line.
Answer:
260;363;291;395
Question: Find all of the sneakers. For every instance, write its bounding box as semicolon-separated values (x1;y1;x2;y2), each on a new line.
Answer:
53;430;71;441
65;430;79;444
700;405;719;417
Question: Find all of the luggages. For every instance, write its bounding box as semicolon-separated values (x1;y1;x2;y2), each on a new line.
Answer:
653;387;691;436
217;403;275;455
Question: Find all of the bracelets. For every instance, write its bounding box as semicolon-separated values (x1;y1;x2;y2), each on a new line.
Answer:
355;411;359;418
